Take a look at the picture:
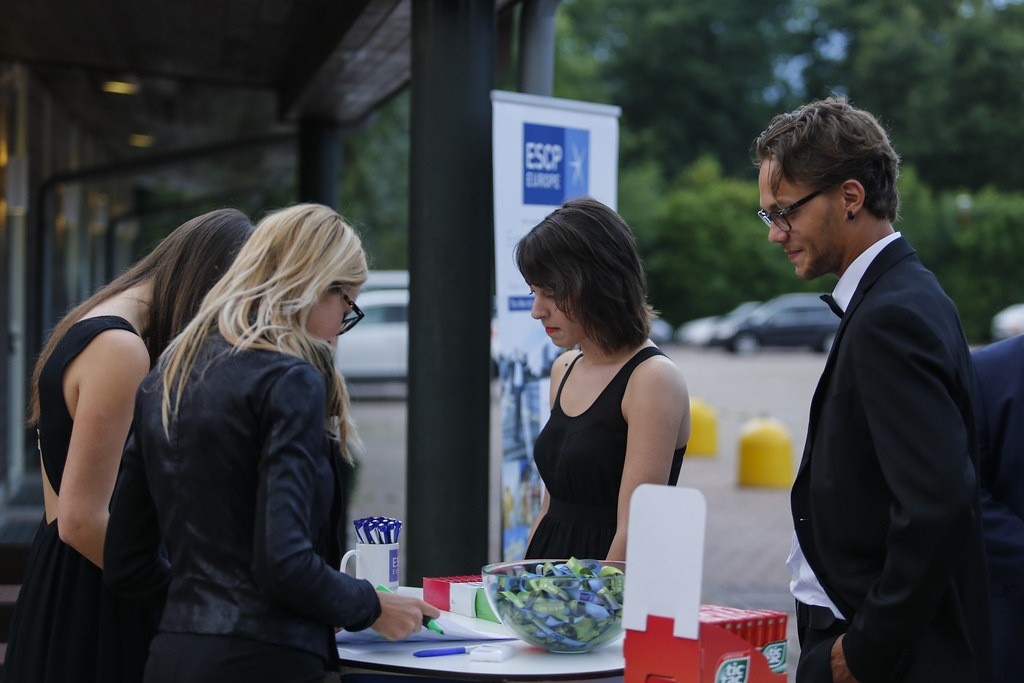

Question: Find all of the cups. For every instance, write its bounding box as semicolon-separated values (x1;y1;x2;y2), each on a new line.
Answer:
339;542;399;593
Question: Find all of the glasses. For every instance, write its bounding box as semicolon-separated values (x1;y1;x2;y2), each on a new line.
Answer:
756;185;822;230
330;287;366;338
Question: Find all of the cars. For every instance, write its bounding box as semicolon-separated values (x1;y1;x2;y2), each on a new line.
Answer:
705;292;842;355
335;288;409;382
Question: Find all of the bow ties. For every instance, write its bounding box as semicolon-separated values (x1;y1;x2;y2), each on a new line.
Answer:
818;292;847;323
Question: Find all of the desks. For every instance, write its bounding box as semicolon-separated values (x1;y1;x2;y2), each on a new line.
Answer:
334;574;626;681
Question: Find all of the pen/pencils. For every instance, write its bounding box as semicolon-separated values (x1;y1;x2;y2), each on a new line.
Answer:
413;644;481;657
353;515;403;545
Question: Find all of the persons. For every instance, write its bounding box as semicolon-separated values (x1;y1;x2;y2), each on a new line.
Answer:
512;195;691;579
0;208;257;683
134;202;441;683
967;330;1024;683
747;86;996;683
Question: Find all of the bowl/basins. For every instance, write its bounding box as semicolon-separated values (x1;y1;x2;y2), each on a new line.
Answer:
481;556;626;655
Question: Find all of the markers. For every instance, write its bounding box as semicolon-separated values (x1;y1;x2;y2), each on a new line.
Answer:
375;584;447;636
